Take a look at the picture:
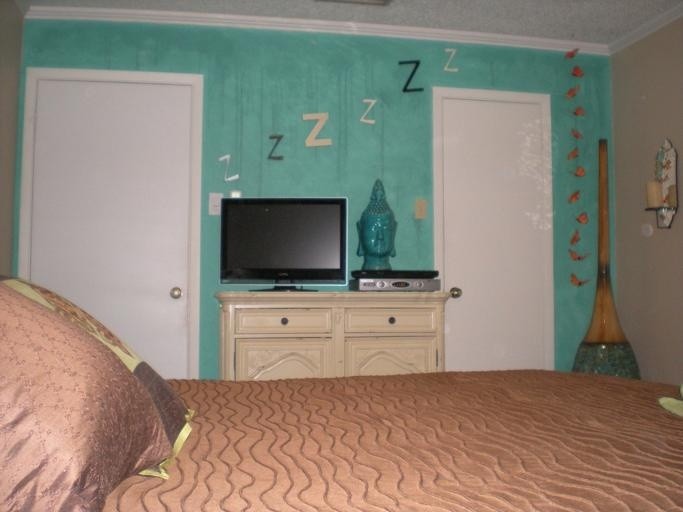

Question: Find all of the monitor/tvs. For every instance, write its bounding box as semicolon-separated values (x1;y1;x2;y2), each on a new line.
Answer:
216;197;349;292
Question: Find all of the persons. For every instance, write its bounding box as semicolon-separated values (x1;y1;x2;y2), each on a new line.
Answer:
356;178;399;270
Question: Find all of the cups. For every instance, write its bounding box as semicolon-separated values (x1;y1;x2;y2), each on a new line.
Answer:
645;182;663;208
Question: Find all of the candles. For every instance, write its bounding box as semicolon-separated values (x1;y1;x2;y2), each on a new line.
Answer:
647;177;663;209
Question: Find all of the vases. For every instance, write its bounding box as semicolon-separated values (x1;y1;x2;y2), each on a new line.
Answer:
572;138;641;380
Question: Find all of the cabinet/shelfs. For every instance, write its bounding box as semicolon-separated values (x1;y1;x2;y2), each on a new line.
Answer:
217;293;450;383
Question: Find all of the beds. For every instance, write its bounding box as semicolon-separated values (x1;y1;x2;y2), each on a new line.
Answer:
103;370;683;511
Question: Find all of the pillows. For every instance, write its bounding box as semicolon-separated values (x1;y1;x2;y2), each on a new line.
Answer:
0;283;177;511
0;276;194;479
